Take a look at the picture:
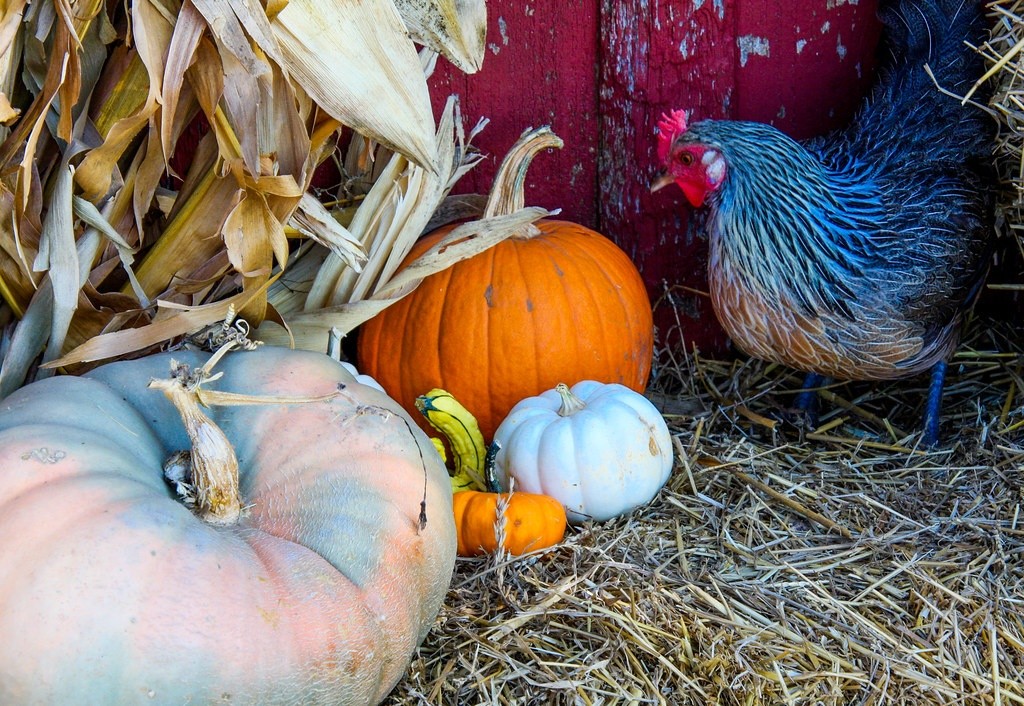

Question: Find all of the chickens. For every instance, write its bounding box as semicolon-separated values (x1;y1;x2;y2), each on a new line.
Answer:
647;1;1001;453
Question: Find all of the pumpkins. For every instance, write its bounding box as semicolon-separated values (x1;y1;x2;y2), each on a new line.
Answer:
0;123;674;706
